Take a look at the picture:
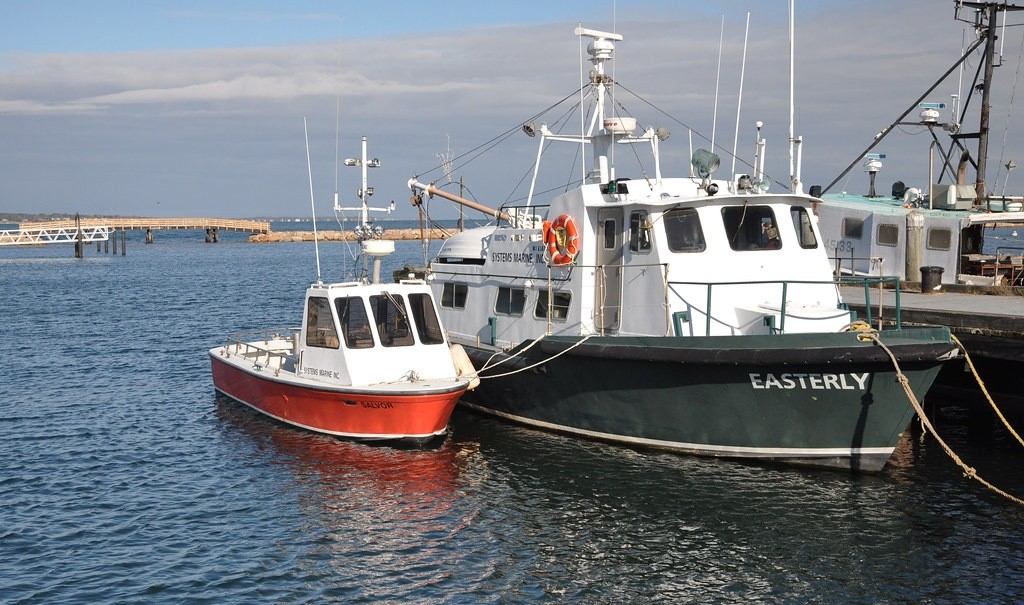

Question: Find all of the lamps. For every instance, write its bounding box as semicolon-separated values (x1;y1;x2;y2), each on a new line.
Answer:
367;158;381;167
707;182;719;196
345;158;361;166
809;186;821;198
367;187;373;196
618;184;629;200
357;189;363;198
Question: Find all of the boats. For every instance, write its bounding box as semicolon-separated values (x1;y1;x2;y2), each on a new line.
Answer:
808;0;1024;284
209;95;470;449
392;1;964;475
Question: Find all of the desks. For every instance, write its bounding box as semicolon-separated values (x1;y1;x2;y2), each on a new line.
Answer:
960;262;1013;286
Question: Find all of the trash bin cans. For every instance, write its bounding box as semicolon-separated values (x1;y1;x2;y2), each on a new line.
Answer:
920;266;944;293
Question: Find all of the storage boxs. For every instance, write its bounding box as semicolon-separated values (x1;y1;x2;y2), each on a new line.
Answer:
932;185;978;211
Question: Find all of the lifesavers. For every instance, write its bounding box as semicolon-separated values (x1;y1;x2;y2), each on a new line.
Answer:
548;214;580;265
